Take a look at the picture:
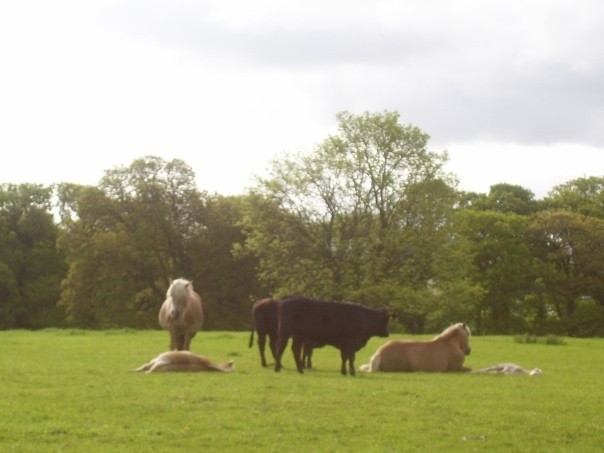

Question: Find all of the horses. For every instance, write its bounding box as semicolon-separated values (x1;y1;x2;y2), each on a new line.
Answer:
359;320;473;373
474;362;542;377
131;348;234;373
157;277;203;350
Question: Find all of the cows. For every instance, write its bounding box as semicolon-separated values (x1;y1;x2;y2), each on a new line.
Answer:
248;294;326;368
272;295;389;376
